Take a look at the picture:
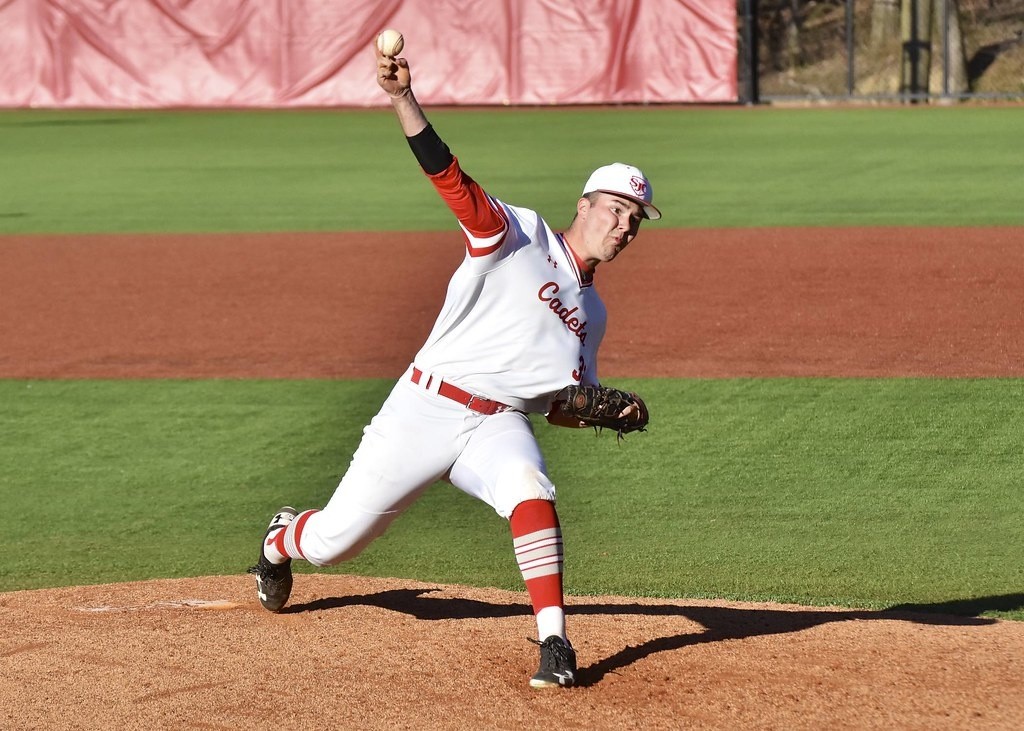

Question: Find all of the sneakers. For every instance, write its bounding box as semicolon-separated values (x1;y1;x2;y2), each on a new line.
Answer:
527;635;578;688
246;506;299;612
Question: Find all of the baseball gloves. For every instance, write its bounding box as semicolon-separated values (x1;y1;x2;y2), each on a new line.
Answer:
545;383;649;434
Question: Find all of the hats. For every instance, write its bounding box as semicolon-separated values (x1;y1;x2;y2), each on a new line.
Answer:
582;162;662;220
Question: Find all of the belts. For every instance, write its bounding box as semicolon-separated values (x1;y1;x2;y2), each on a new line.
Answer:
411;367;529;416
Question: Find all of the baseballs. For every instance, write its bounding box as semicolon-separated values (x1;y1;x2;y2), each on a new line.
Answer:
377;28;404;56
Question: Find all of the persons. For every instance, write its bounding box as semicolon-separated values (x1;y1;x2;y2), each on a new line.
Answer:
252;30;663;691
